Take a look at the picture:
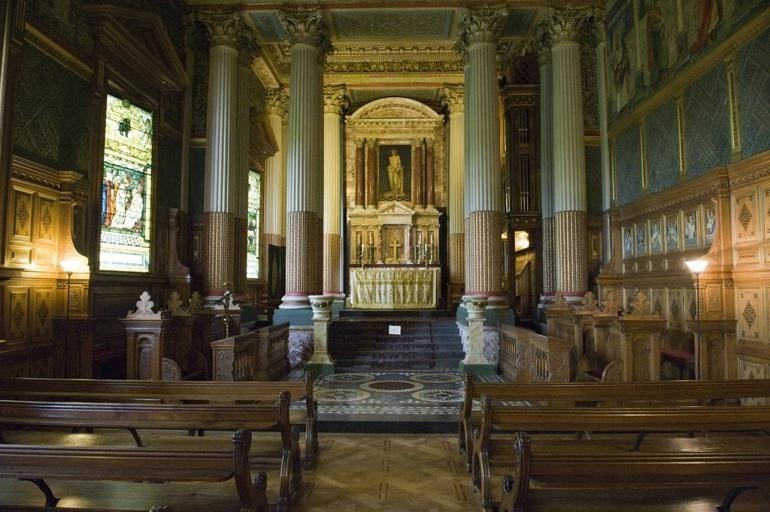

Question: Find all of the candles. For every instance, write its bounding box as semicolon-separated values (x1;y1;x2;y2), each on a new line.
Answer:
358;234;361;244
425;244;428;253
430;234;433;244
369;233;372;245
361;244;364;253
419;235;422;243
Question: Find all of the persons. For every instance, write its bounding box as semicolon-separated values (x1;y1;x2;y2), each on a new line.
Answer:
101;172;144;232
386;148;402;190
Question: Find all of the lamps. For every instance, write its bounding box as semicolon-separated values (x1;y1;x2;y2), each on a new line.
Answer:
686;260;709;380
61;261;78;320
